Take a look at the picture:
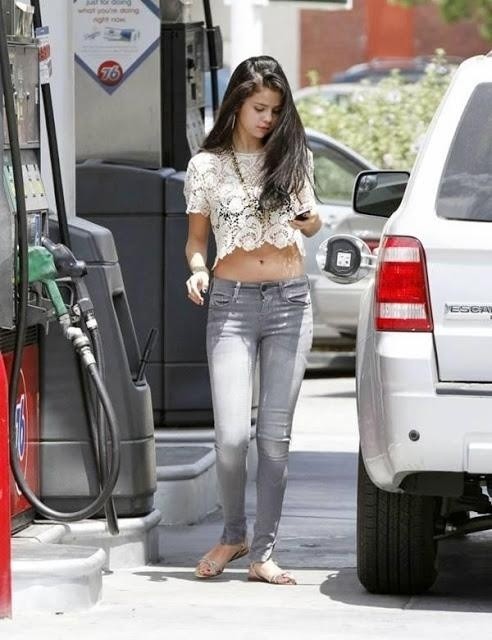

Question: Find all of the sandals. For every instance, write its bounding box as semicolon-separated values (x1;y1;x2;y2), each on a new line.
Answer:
194;536;250;579
248;561;297;586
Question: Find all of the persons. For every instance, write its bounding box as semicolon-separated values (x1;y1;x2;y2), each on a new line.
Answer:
183;52;322;590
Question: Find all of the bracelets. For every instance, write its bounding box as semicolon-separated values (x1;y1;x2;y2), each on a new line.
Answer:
192;266;210;274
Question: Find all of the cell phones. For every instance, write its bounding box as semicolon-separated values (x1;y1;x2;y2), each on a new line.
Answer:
293;206;312;220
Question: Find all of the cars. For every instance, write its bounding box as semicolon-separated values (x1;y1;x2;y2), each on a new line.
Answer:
200;119;404;369
352;51;492;595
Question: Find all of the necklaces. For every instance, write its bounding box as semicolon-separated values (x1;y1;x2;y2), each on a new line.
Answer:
227;139;273;226
